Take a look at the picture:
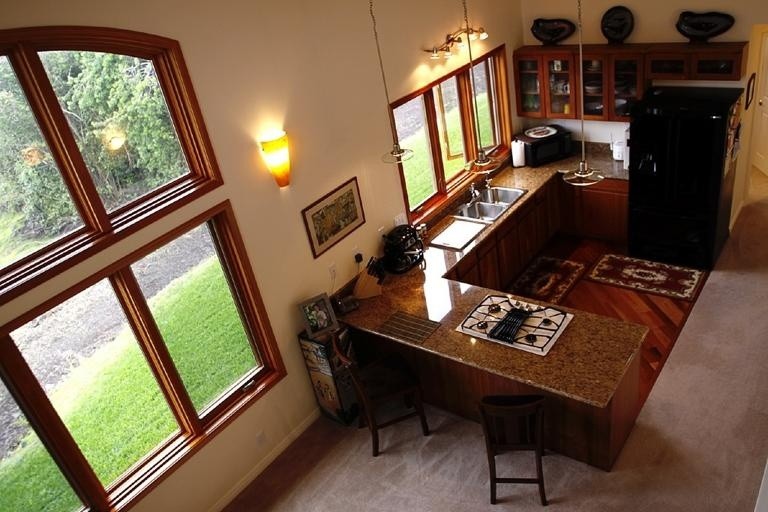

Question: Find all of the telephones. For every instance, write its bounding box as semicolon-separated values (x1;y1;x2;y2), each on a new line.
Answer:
335;293;360;315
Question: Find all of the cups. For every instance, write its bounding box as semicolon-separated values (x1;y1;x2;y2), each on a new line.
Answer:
563;84;570;94
591;59;600;66
554;83;563;94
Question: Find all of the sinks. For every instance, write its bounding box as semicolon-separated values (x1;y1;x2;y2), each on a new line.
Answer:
457;202;505;221
477;188;522;202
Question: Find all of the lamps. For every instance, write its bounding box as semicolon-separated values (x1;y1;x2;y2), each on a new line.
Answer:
562;1;605;186
369;0;415;165
424;28;488;60
462;1;502;174
257;131;291;187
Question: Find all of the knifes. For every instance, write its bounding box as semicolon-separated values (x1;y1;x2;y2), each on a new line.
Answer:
366;256;387;285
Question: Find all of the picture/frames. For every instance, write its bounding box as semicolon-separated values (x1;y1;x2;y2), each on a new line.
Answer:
745;73;756;109
301;176;366;259
297;292;339;339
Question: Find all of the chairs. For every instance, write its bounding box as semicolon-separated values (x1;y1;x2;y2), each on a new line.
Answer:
332;334;429;456
477;393;546;505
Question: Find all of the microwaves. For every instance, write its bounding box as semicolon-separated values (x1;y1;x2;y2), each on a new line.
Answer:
514;123;573;169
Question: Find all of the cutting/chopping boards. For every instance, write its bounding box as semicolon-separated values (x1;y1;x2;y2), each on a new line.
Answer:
430;219;486;252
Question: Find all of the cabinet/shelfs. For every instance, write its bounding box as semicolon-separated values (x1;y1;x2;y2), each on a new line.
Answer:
297;320;360;427
447;172;636;274
513;41;750;123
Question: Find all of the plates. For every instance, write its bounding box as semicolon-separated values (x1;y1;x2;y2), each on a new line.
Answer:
525;125;558;139
586;101;600;111
584;85;601;94
615;99;626;110
585;65;602;71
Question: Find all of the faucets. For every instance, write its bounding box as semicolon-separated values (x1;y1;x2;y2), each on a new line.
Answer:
484;175;493;191
470;183;480;200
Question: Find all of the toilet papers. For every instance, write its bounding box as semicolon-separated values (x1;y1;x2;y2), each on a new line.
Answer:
512;141;525;167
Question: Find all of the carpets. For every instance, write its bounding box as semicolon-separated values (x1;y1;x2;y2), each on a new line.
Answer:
582;249;706;302
506;255;585;304
